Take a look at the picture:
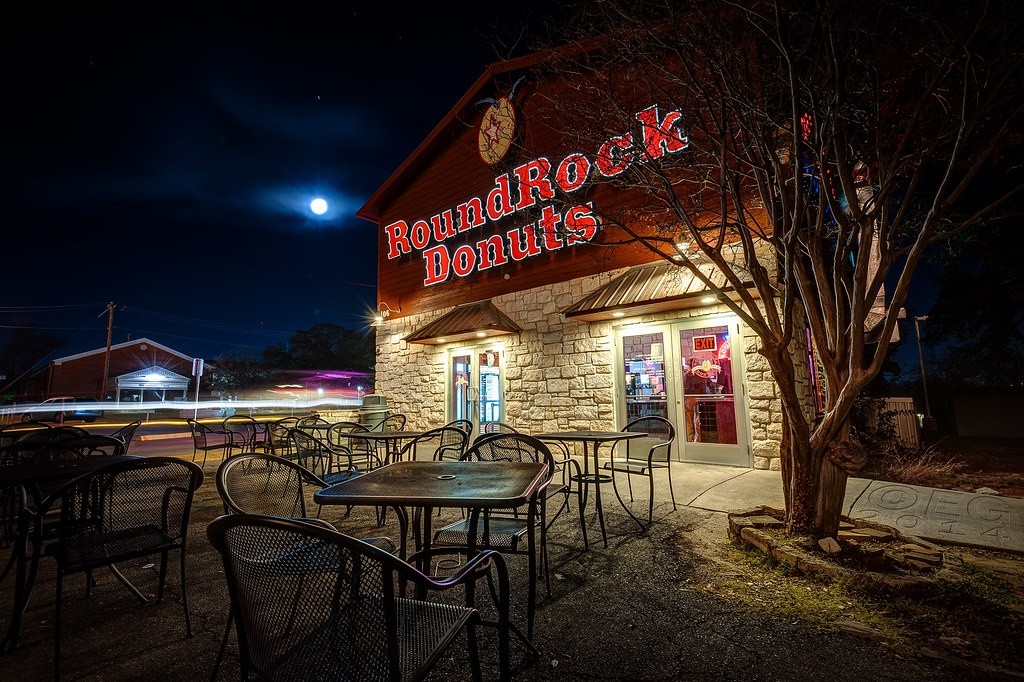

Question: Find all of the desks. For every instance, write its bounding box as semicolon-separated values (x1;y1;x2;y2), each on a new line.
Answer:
0;453;170;604
297;424;373;474
314;460;549;682
340;431;441;466
229;420;293;475
533;430;648;550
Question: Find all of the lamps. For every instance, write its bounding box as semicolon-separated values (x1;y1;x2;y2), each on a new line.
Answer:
370;302;401;326
672;229;701;261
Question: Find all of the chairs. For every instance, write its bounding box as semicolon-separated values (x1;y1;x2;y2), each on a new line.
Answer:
0;414;676;682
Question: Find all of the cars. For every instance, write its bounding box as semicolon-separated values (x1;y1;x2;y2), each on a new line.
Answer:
21;397;103;424
